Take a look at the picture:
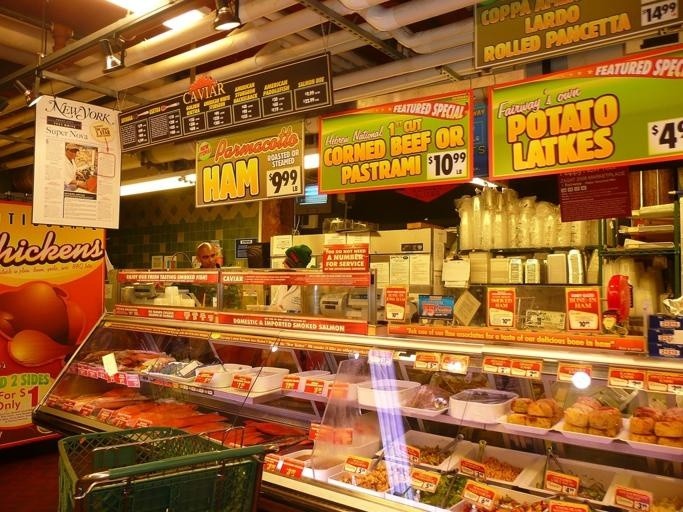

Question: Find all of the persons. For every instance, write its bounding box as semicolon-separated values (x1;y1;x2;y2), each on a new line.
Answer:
64;144;88;192
195;242;221;303
270;244;313;313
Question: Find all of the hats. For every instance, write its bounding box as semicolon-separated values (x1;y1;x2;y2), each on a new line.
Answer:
286;245;312;267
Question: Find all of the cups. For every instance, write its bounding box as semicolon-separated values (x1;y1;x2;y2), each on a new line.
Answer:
601;258;662;315
458;188;592;250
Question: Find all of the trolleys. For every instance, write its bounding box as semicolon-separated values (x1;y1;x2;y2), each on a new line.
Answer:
55;426;266;511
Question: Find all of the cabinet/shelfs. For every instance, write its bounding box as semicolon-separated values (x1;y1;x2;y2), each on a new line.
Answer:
30;318;682;511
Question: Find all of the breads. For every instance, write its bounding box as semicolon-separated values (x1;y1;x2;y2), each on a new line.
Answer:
508;397;683;448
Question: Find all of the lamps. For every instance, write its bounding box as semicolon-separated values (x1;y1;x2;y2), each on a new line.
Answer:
97;30;125;75
212;0;241;33
13;67;46;109
